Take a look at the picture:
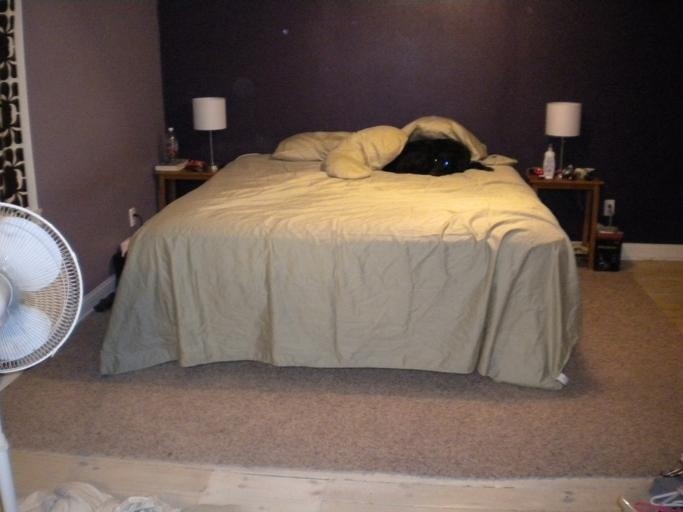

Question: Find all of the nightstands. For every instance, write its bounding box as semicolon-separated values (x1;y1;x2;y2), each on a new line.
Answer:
153;164;217;214
522;169;606;272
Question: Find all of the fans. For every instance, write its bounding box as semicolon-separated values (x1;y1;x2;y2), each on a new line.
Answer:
0;202;85;377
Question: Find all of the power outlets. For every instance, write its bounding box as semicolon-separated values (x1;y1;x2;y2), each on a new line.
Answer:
128;207;137;228
603;199;615;217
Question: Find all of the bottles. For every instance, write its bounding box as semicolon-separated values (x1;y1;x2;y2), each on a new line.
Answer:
543;144;556;179
163;127;178;162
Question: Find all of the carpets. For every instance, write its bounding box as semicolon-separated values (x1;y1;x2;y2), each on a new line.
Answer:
0;257;682;480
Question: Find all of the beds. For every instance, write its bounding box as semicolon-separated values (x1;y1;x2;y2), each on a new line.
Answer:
97;150;583;393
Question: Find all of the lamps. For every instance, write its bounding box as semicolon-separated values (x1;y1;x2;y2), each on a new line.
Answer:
191;96;228;173
544;100;582;180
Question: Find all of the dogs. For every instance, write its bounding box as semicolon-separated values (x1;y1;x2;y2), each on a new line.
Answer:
383;139;494;176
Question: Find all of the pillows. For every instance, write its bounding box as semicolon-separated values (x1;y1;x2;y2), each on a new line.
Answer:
320;124;409;180
400;114;489;164
271;130;352;162
